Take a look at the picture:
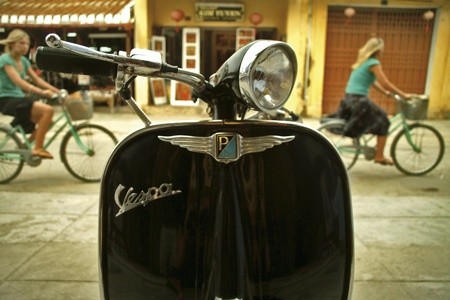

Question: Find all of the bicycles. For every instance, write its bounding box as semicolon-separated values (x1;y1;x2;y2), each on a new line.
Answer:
317;93;446;177
0;89;119;183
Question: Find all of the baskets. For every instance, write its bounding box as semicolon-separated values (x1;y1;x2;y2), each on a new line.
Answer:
66;97;93;120
404;95;429;120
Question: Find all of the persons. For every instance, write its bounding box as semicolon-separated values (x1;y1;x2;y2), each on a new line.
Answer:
0;29;61;159
320;37;417;166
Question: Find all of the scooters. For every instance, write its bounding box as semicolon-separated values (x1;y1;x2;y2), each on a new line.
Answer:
34;33;355;300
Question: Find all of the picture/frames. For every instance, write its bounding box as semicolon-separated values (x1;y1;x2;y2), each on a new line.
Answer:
214;27;256;51
149;27;201;107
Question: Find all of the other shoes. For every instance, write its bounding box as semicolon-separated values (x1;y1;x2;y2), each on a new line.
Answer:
31;148;53;158
375;158;394;165
